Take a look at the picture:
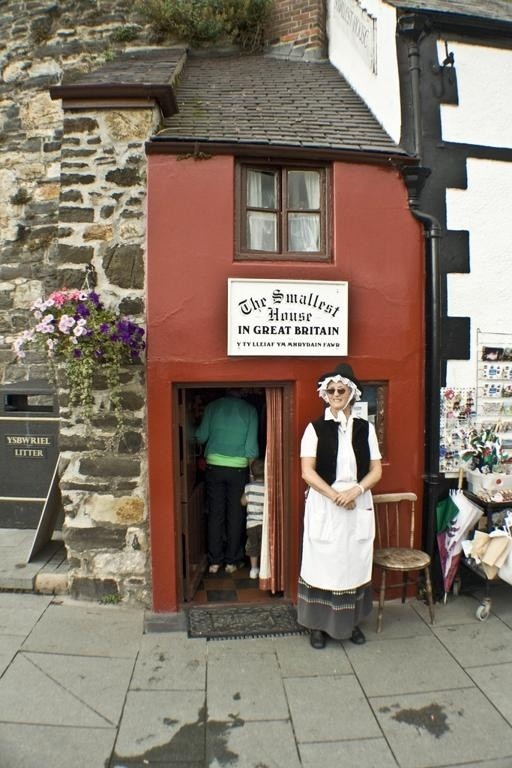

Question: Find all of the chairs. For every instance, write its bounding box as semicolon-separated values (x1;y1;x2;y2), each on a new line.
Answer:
371;490;438;633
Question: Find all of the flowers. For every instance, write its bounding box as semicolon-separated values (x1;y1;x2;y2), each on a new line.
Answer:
10;288;147;457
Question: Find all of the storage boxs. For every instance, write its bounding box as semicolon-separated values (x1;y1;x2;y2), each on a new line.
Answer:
466;465;511;503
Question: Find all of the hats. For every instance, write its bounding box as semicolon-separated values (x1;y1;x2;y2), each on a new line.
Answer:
317;362;362;404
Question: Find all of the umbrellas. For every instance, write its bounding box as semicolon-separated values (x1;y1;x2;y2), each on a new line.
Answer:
435;467;485;605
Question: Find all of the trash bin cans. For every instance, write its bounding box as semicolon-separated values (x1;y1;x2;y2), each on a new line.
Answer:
0;378;64;566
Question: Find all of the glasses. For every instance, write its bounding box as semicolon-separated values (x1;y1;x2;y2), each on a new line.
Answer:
322;386;350;395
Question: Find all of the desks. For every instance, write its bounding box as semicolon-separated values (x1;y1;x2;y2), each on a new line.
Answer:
462;487;512;537
458;557;511;620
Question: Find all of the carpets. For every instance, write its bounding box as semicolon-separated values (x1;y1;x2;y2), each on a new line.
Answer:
180;594;306;640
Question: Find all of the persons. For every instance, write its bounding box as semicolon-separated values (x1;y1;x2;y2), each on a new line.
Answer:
240;459;265;580
194;390;260;575
295;363;383;649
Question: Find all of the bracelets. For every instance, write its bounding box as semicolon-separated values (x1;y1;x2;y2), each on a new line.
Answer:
355;484;364;495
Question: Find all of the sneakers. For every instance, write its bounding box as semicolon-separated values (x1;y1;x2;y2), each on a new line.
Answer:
249;569;260;578
208;564;221;574
225;562;246;574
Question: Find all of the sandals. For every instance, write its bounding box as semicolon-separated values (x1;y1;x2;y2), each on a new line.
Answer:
310;627;326;649
351;628;365;644
